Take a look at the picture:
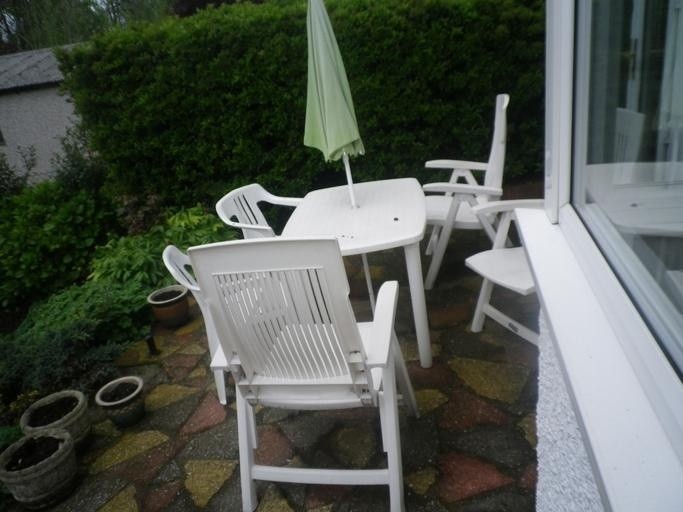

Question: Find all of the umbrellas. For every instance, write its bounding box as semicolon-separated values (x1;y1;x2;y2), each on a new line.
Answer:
303;0;376;319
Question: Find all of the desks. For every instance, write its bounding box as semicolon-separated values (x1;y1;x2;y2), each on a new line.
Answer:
582;161;683;237
280;177;432;369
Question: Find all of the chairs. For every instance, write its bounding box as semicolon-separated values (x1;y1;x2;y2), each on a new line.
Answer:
218;183;303;240
188;237;417;512
422;94;510;285
609;108;647;246
466;199;541;345
162;245;232;405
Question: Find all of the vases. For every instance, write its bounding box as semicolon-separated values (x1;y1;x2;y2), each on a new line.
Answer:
97;375;146;429
0;428;81;507
20;390;95;448
146;285;192;330
